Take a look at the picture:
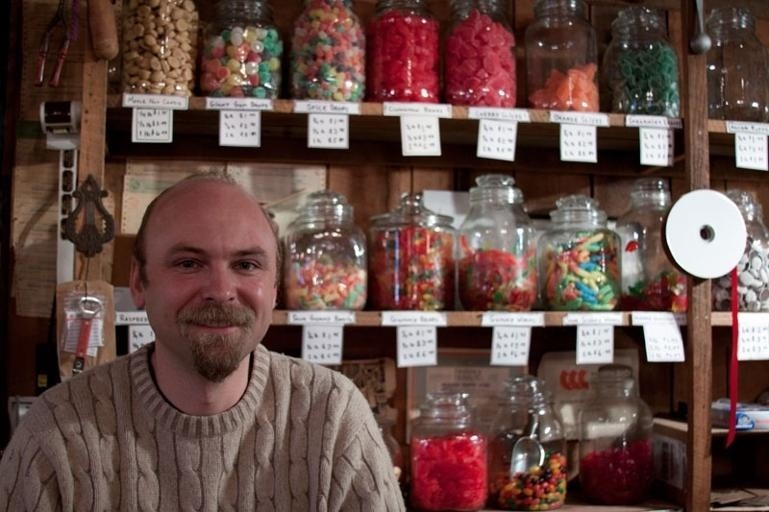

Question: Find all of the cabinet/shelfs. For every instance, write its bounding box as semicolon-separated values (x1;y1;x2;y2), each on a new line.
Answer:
69;1;768;511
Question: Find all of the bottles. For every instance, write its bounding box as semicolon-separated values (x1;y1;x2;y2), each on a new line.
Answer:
367;0;443;103
284;0;367;102
490;373;569;512
711;186;769;314
616;175;690;313
457;167;539;312
444;0;518;105
706;5;767;123
366;184;456;310
539;191;623;312
409;390;490;512
601;9;682;120
372;413;407;492
199;3;284;100
575;363;656;504
280;188;370;310
524;1;600;113
122;1;201;98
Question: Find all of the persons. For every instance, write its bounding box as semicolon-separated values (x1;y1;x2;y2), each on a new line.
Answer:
0;170;409;512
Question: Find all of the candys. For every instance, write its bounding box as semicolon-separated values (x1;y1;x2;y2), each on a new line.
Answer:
121;1;769;512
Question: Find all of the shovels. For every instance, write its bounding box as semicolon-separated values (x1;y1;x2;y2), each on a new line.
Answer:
510;414;546;474
